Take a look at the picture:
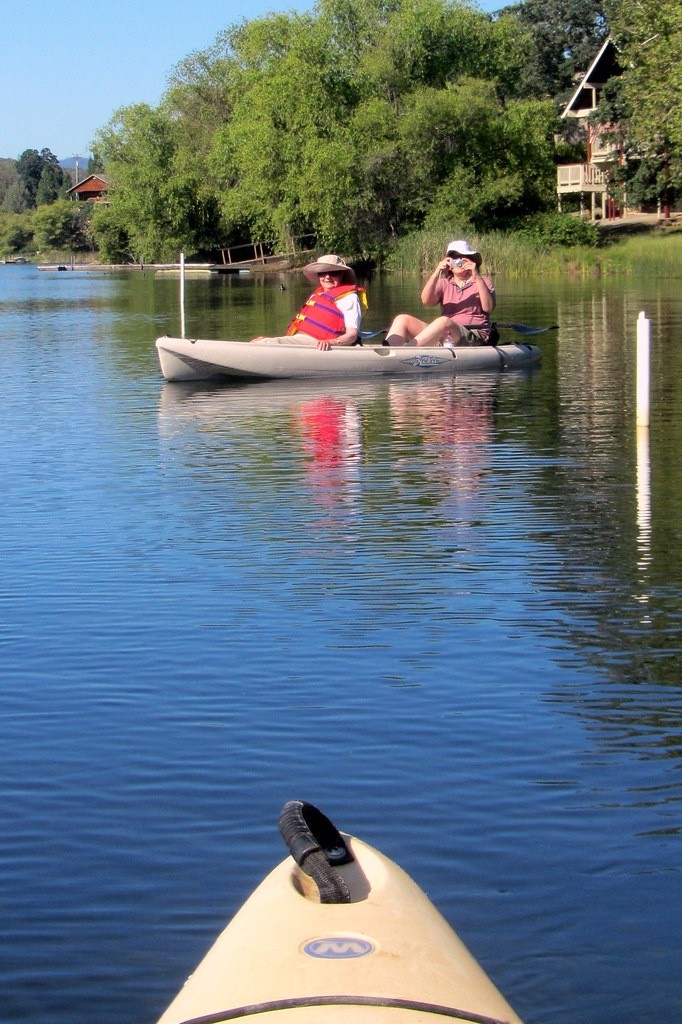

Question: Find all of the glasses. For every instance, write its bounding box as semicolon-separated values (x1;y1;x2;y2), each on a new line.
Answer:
318;271;337;277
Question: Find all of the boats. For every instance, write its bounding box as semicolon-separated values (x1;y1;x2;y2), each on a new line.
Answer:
156;252;546;384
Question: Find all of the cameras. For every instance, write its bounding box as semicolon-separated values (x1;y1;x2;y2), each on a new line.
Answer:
450;259;465;268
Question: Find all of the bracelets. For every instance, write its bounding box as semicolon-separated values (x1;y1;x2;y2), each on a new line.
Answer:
336;338;341;346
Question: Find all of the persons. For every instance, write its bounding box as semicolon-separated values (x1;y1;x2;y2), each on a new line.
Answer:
382;240;496;347
248;254;362;351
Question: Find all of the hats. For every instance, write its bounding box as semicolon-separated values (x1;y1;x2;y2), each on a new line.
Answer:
446;240;482;266
303;254;355;284
